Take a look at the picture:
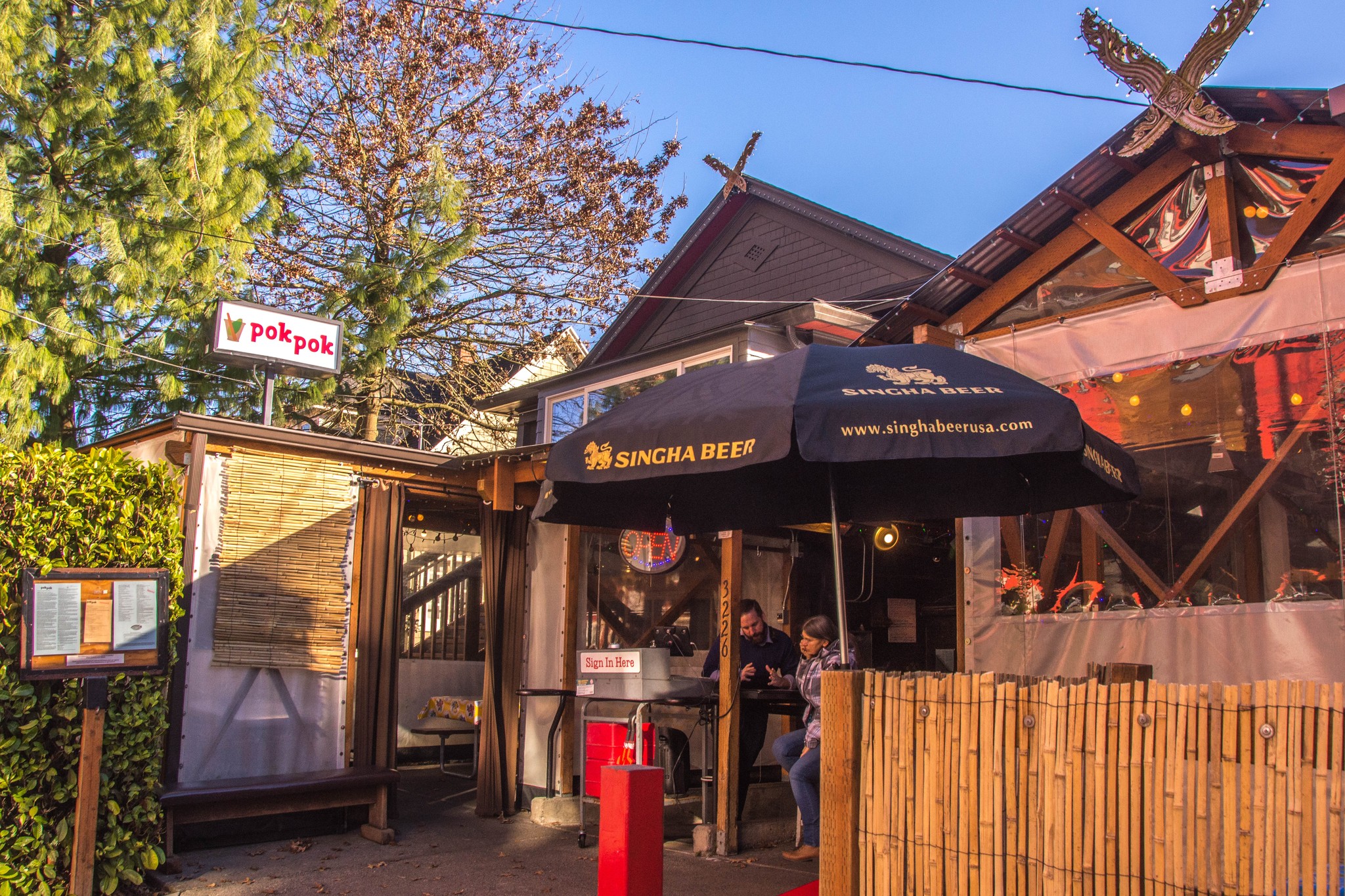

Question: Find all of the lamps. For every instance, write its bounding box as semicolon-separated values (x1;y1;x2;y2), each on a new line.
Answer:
872;522;899;551
1207;355;1235;473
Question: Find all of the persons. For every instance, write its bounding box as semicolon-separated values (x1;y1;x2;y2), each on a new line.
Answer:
701;600;798;820
772;615;861;860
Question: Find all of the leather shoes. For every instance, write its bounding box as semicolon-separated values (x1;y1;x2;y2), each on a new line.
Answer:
782;846;820;863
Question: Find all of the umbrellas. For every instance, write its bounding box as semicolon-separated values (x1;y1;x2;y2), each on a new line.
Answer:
530;342;1140;668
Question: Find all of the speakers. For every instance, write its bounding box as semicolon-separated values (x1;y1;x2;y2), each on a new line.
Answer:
656;728;690;794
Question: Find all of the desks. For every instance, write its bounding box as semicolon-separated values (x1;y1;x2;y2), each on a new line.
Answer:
577;696;806;849
418;696;483;779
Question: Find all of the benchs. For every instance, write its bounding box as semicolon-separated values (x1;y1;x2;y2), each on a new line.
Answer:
411;727;481;779
